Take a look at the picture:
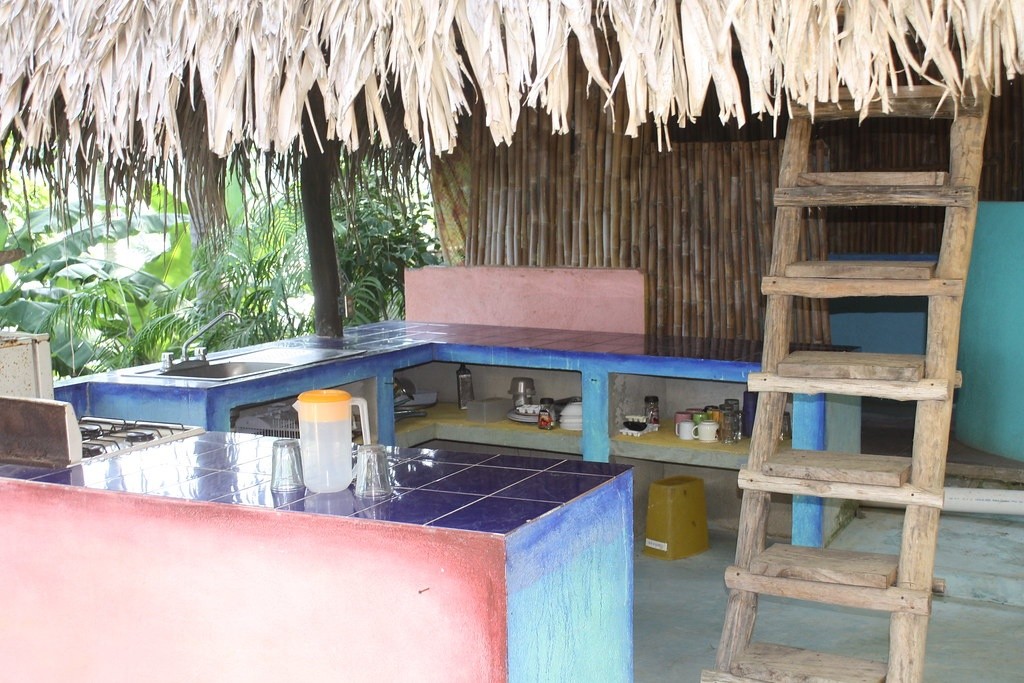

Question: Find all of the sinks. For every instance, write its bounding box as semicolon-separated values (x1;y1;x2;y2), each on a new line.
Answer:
119;346;370;383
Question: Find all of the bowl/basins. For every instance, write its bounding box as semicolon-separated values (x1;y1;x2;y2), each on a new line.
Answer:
508;376;537;395
559;400;583;431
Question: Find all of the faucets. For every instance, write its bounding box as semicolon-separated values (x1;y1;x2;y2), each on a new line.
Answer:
180;310;243;362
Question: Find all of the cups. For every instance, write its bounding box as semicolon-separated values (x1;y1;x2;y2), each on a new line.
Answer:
722;412;738;447
724;398;739;412
355;444;393;499
742;391;760;439
703;405;718;420
271;438;306;494
732;410;746;441
692;412;710;436
675;420;695;440
710;408;720;436
692;420;720;443
685;407;703;419
718;404;735;414
675;412;692;432
781;412;792;439
513;393;532;407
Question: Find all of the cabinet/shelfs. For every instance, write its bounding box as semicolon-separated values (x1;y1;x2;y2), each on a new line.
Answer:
206;341;433;450
434;325;863;548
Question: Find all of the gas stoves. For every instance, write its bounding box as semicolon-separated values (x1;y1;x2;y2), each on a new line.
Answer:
78;415;207;463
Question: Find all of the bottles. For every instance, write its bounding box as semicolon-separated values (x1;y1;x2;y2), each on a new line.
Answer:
645;396;659;425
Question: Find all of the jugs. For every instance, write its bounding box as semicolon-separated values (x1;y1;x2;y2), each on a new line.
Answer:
292;389;370;493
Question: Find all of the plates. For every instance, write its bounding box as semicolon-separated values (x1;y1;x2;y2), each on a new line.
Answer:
505;405;562;422
